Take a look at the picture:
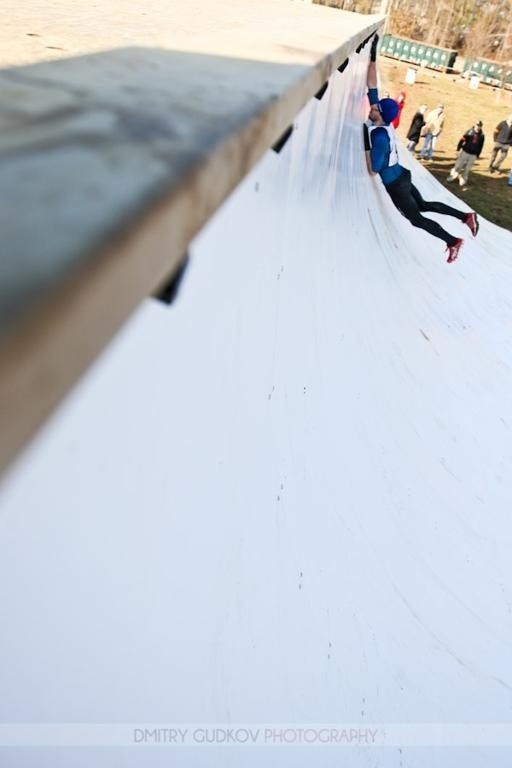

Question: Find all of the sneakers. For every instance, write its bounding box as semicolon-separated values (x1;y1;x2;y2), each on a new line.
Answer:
447;238;463;263
446;174;469;187
466;212;479;236
488;165;500;174
418;154;434;164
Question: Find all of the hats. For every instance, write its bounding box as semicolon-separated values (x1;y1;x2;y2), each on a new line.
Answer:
476;121;483;127
378;98;399;125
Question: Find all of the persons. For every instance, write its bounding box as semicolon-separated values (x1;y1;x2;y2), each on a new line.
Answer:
363;33;478;263
406;104;428;152
419;104;445;159
391;93;406;129
446;121;484;186
487;115;512;175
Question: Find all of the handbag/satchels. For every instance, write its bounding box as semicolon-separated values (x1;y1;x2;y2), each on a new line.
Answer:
420;123;430;137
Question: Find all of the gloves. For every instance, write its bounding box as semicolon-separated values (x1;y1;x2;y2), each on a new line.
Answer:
370;34;378;62
363;122;372;153
